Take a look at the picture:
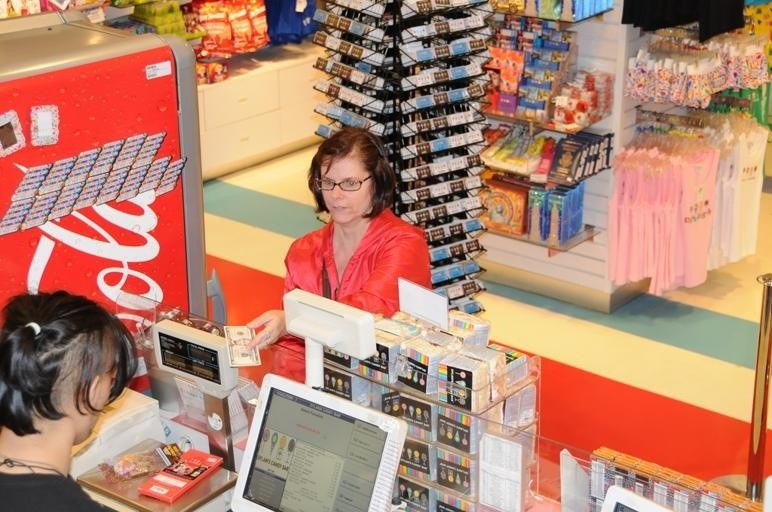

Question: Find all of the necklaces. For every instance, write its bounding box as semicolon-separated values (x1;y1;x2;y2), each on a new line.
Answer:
0;458;71;476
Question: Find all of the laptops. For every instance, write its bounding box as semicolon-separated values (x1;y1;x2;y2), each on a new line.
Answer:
218;372;408;512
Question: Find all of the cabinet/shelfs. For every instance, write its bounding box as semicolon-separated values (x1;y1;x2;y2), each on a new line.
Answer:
311;1;487;315
476;0;769;315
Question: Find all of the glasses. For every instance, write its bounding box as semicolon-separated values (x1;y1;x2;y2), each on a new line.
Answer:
313;175;373;191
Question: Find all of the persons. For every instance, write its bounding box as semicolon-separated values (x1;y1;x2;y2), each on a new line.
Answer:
246;129;431;354
0;289;166;512
